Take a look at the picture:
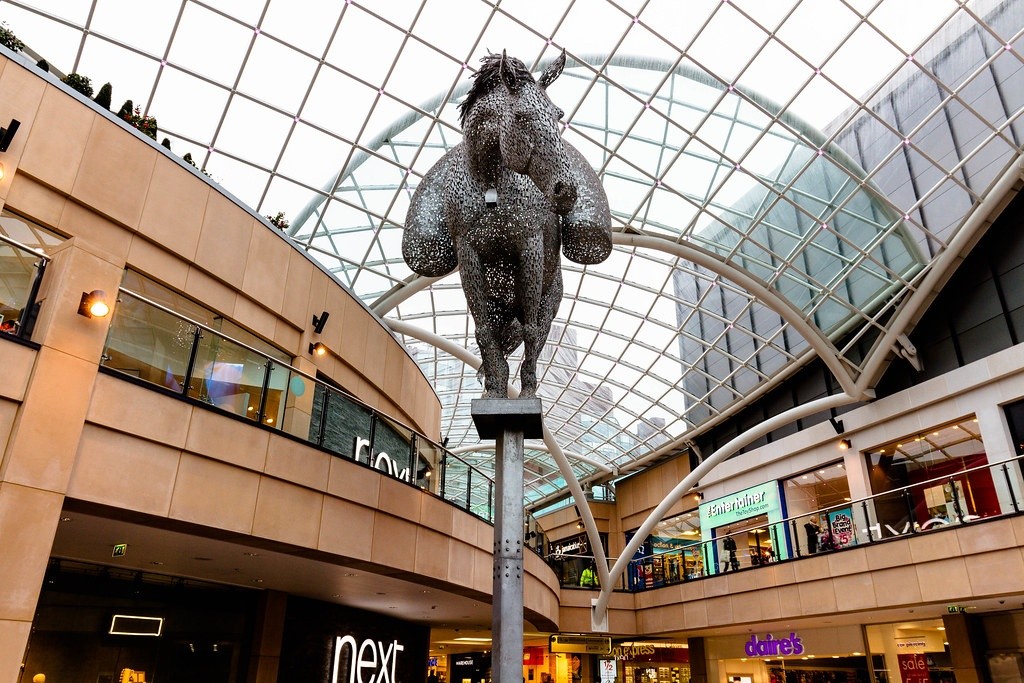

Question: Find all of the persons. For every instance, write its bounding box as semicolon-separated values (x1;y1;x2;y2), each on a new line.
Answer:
427;670;440;683
804;516;821;554
580;564;599;587
723;532;738;572
0;308;25;335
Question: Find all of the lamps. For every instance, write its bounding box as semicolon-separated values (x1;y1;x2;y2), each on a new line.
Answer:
695;492;704;501
840;439;852;449
309;342;326;356
312;312;330;335
77;287;111;318
0;119;21;153
417;466;433;480
577;522;586;529
246;402;277;423
526;531;536;539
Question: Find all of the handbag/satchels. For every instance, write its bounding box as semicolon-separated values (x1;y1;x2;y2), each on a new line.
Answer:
720;549;730;562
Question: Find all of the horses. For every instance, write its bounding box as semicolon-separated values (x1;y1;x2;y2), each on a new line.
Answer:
456;45;578;399
